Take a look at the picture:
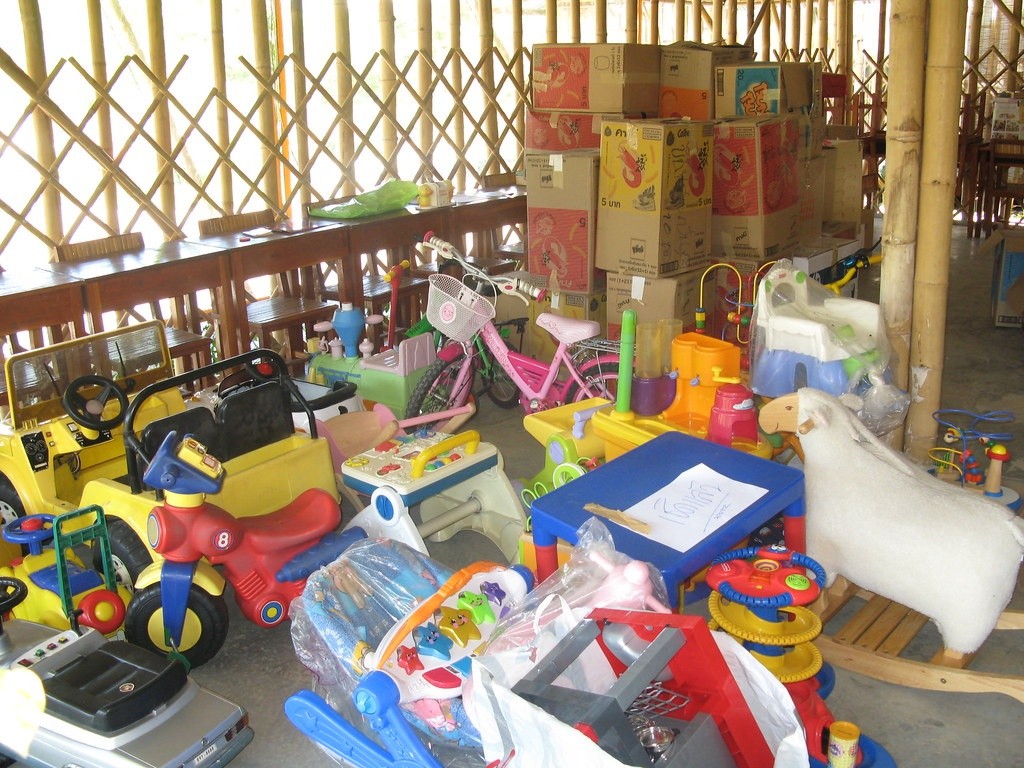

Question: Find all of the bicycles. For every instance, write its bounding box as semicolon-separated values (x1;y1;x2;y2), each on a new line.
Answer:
403;228;638;433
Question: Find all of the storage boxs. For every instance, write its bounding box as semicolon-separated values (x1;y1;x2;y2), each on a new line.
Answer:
463;36;1024;379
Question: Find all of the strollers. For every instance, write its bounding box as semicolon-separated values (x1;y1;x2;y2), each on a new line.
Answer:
275;525;565;767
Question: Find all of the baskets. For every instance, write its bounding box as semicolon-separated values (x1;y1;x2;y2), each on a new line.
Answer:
426;273;497;342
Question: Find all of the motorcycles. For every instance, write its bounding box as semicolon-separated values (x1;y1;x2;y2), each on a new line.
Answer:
122;431;372;670
